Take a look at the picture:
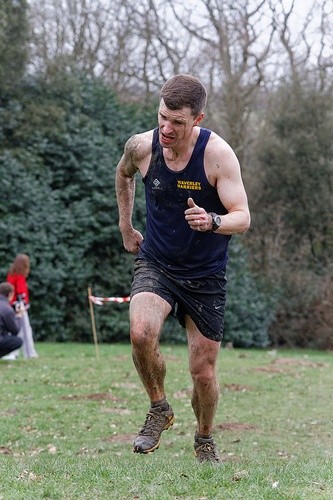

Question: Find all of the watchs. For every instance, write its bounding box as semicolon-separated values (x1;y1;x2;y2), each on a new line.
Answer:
208;212;221;234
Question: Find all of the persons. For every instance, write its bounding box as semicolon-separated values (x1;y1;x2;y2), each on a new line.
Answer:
0;282;23;361
115;72;251;464
6;254;38;359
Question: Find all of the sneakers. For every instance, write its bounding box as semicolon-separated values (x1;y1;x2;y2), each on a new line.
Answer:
194;437;221;463
134;405;174;454
